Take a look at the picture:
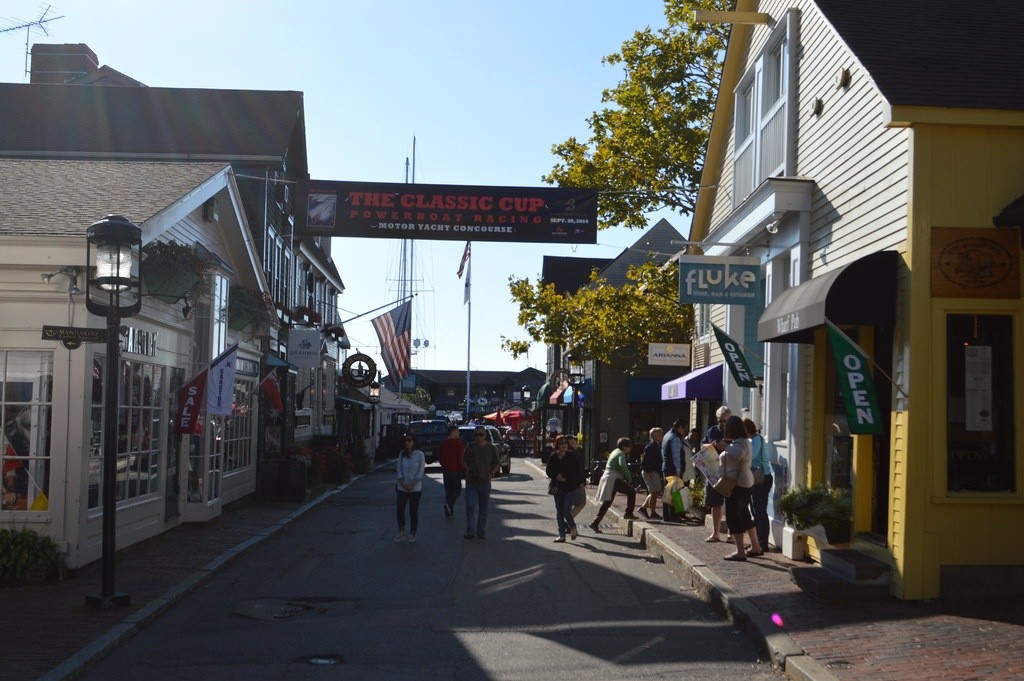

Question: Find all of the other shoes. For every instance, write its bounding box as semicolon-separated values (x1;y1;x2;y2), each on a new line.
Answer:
637;507;649;518
589;523;602;533
745;548;764;557
393;535;405;542
464;534;484;539
553;536;565;542
744;544;769;551
571;527;577;540
649;512;663;520
444;504;450;519
408;533;416;542
624;513;639;519
726;537;735;543
724;553;747;561
705;534;720;542
450;512;455;518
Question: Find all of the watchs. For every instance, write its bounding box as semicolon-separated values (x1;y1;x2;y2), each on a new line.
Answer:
490;472;495;478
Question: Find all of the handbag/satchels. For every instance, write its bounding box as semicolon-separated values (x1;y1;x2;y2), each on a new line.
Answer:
713;475;736;498
547;480;559;495
662;476;694;513
751;466;764;485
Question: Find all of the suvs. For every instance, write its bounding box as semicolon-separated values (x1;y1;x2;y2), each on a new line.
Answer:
454;426;512;476
404;419;450;466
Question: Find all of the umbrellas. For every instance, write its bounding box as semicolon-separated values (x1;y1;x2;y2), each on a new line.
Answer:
483;406;534;426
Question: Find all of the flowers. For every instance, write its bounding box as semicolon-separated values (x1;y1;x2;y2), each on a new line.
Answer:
298;306;322;323
284;446;354;480
142;240;218;305
689;482;704;509
229;285;275;329
333;326;345;337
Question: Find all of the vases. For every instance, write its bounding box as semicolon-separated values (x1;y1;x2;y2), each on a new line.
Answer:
312;321;317;325
331;333;335;338
300;313;308;322
338;336;343;342
689;508;711;521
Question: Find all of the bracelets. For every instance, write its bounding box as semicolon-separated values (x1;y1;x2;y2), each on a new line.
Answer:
7;485;16;491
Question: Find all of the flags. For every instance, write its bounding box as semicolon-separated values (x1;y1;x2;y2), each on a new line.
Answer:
371;300;412;386
456;241;472;303
260;368;283;414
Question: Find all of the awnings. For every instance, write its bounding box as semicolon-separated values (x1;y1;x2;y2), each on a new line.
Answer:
756;250;899;343
549;386;573;405
660;364;723;401
334;396;374;411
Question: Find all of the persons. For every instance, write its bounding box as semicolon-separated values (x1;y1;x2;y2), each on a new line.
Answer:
588;405;773;561
546;434;587;543
392;434;425;543
0;428;18;507
438;425;501;540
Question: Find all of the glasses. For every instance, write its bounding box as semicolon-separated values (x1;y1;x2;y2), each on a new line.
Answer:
475;434;484;436
717;420;726;423
404;439;411;442
558;441;567;445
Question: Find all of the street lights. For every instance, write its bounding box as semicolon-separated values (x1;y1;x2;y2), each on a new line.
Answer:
83;213;145;614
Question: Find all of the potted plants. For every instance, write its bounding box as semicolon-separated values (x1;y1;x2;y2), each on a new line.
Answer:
775;481;853;544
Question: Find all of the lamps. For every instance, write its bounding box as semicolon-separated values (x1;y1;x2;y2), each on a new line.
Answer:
638;284;679;304
766;212;784;233
133;293;192;319
41;267;80;294
204;198;220;223
